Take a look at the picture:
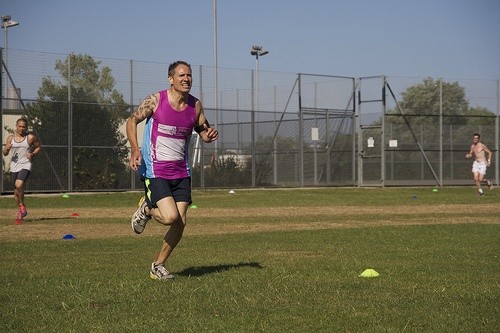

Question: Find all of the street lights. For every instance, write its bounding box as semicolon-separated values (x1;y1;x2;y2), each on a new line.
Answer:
0;15;20;108
250;45;270;188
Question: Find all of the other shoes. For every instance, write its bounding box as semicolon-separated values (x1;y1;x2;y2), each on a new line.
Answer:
487;179;494;189
478;188;484;195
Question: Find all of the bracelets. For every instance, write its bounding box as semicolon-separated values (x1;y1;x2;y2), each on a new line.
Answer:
31;152;34;157
488;159;491;162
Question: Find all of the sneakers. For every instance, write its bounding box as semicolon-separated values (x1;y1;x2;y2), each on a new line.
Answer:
16;204;27;225
149;262;175;281
131;196;152;233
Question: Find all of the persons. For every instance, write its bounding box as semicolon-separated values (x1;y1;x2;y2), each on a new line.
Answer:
127;60;219;281
3;118;41;225
465;133;494;196
